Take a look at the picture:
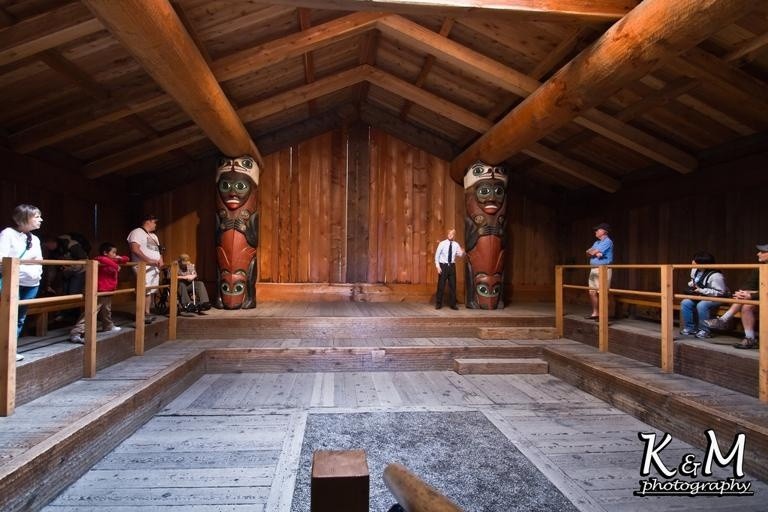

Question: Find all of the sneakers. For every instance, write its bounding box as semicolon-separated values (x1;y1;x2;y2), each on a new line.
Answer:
680;328;698;335
201;302;211;308
105;325;122;334
70;333;84;343
703;317;728;330
696;330;711;338
733;337;758;348
188;303;195;311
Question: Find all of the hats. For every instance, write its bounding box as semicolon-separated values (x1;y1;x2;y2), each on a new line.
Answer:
756;243;768;251
179;255;190;264
593;223;611;232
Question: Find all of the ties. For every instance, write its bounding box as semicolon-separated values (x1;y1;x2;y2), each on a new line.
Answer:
448;242;452;262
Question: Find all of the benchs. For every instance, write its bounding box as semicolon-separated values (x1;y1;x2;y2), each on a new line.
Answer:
18;290;136;332
608;293;758;331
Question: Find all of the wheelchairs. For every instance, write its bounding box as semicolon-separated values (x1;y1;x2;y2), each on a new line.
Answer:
154;262;202;314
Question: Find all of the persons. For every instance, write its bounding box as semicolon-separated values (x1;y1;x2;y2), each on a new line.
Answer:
0;202;46;364
464;163;509;309
705;244;768;349
127;215;166;325
68;242;124;343
212;154;261;311
433;227;467;311
676;250;735;340
42;233;89;328
583;221;617;324
166;254;213;313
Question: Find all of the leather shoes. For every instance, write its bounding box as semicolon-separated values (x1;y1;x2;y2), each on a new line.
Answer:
436;305;440;309
584;314;599;319
451;306;457;309
